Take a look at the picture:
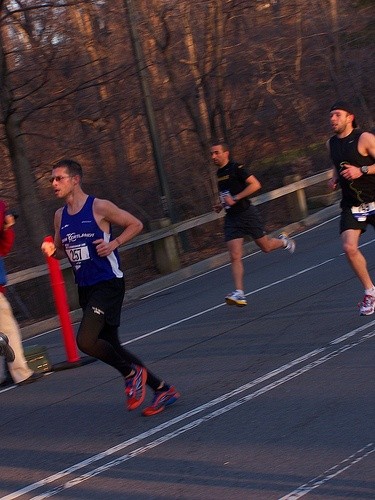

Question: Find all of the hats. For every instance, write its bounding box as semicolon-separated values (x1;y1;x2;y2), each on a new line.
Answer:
329;102;357;129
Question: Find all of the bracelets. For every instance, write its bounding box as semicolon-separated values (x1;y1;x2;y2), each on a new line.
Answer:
116;238;121;245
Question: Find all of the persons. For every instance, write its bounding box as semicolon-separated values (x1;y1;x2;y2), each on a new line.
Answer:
0;197;44;388
40;158;182;418
324;101;375;317
208;140;297;309
0;332;15;364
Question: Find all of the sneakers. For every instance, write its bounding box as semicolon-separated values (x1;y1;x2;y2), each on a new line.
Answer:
277;232;296;254
356;289;375;316
141;381;181;416
225;291;248;308
124;364;148;410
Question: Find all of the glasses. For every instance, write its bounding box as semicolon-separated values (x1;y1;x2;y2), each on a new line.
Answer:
49;175;74;183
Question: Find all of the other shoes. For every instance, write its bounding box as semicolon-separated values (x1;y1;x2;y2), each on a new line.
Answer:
17;372;44;385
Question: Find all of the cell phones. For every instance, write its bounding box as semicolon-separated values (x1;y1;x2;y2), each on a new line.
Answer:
339;166;348;171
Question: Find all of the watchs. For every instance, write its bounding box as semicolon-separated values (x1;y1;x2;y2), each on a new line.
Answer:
361;165;368;175
232;196;238;202
